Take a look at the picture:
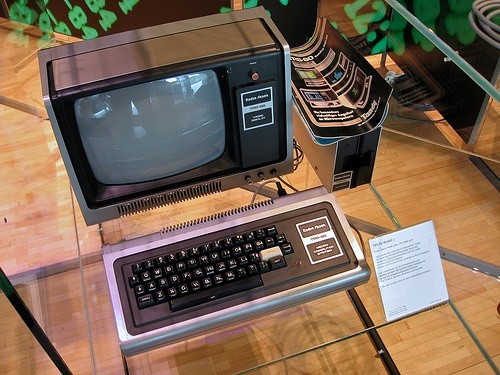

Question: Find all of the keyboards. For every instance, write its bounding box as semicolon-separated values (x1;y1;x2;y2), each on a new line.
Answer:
101;187;371;358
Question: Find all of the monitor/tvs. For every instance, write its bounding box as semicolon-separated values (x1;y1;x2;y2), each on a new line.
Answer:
37;5;293;227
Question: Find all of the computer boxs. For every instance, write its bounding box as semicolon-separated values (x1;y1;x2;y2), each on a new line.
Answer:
293;105;382;192
